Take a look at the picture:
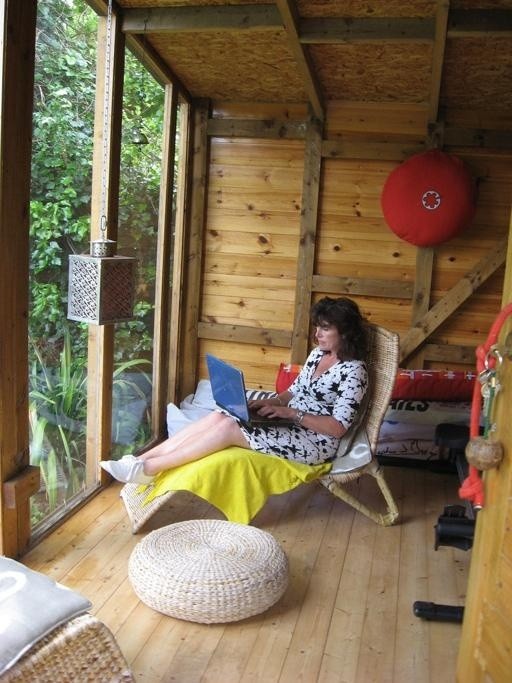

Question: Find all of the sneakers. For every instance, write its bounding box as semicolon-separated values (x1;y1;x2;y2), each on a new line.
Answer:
100;454;156;485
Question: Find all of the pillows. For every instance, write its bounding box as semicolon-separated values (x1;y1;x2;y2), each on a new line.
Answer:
381;149;479;247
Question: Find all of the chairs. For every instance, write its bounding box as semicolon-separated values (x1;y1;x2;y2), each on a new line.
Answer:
0;556;137;682
119;318;403;535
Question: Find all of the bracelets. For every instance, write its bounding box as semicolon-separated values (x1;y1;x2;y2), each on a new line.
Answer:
269;396;283;403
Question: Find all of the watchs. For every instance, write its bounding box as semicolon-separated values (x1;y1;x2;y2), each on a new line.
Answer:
294;408;305;424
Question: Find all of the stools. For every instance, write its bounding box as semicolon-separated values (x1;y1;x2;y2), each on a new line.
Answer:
127;518;291;625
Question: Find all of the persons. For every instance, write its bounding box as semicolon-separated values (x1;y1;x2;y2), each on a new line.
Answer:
96;295;373;486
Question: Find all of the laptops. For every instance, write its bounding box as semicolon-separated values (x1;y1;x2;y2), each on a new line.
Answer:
205;353;294;426
